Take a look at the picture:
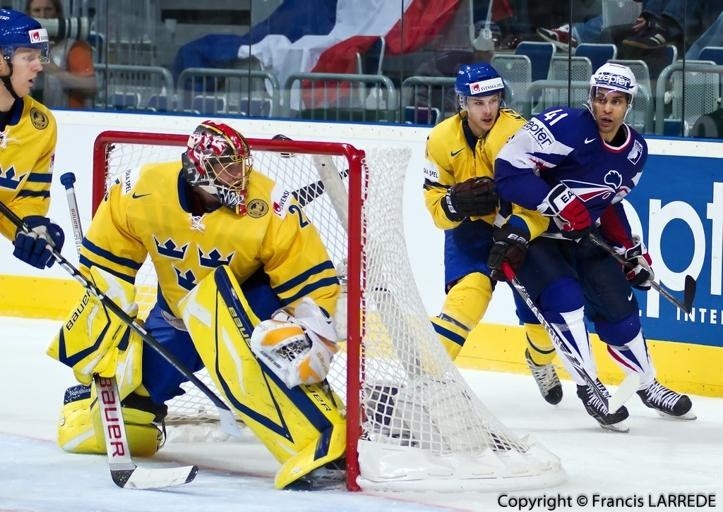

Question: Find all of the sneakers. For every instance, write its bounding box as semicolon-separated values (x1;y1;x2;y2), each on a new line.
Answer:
623;21;673;50
636;378;692;416
575;378;628;424
536;25;582;55
525;348;562;404
307;451;359;481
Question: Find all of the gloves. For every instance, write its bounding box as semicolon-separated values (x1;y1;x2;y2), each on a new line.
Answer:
488;224;529;281
620;236;654;292
439;175;499;222
12;215;64;270
538;184;594;241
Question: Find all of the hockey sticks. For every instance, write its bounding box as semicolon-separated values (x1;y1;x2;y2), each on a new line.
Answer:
0;198;201;489
0;172;242;490
587;232;696;314
500;261;639;414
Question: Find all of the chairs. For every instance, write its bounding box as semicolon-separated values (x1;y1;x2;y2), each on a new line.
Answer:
0;0;722;141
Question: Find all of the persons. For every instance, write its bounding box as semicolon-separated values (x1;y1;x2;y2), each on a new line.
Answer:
1;8;64;270
24;1;98;112
423;59;562;404
473;1;723;141
494;63;692;425
58;120;347;491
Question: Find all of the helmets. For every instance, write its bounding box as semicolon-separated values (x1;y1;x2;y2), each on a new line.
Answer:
453;62;505;102
587;63;638;123
181;120;252;217
0;8;50;65
